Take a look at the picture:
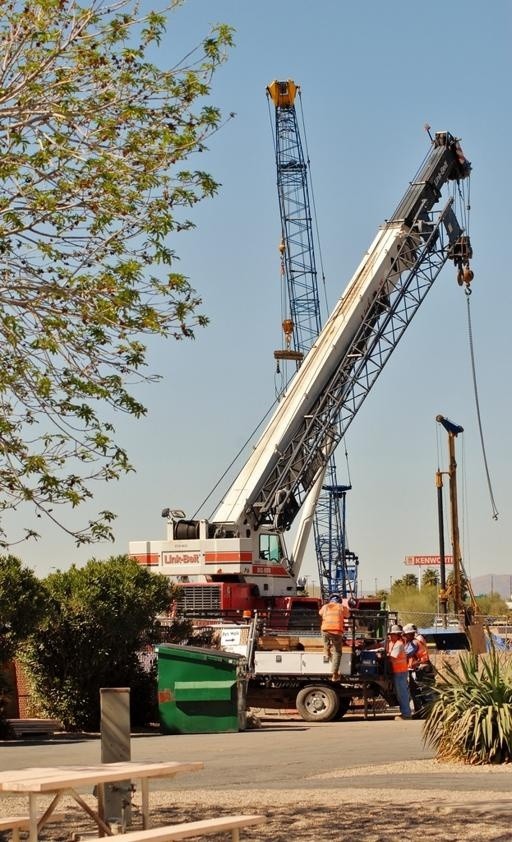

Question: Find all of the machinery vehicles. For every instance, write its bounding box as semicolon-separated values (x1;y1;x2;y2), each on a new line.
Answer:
261;79;358;712
408;412;507;658
128;122;500;723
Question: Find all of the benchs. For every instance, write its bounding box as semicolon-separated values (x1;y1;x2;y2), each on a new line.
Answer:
75;815;266;842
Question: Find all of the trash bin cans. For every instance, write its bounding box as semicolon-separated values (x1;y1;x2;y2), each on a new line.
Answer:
157;642;248;735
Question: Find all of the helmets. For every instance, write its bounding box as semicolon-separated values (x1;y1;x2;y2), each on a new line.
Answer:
329;593;342;603
388;623;417;635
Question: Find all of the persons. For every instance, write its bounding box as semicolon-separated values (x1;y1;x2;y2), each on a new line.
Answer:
387;622;434;721
318;593;350;682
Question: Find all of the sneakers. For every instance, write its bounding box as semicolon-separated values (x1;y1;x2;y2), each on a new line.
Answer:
394;714;412;720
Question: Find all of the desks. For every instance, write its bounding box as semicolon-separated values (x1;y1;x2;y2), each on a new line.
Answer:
0;762;204;842
